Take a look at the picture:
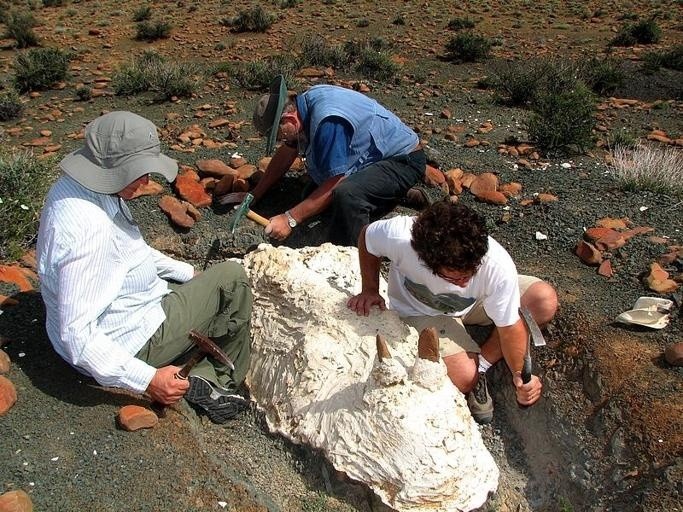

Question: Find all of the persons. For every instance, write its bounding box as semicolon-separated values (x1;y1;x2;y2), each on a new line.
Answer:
34;110;255;426
215;72;427;244
346;197;559;426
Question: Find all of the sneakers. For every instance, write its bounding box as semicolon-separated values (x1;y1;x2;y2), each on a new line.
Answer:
182;375;248;425
469;373;494;425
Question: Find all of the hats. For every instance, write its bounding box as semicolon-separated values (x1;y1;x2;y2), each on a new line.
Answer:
58;111;180;196
252;74;289;158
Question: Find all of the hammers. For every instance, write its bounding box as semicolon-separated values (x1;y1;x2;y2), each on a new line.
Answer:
517;306;545;410
225;193;271;235
148;329;234;419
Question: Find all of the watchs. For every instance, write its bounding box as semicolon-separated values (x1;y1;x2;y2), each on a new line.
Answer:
284;210;297;229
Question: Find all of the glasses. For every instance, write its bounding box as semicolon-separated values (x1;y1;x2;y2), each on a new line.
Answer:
279;129;288;144
